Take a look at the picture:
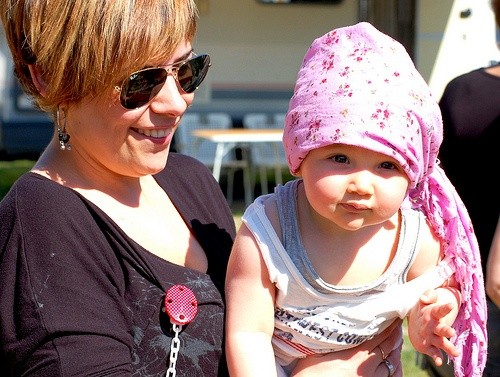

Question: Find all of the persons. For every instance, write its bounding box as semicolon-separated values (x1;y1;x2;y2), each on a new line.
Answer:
0;1;403;377
438;0;500;377
224;22;488;377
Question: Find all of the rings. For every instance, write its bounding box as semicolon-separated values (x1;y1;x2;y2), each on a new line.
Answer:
383;359;395;377
378;345;387;360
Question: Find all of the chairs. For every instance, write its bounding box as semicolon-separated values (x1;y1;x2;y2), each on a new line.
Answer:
174;111;249;206
236;109;298;203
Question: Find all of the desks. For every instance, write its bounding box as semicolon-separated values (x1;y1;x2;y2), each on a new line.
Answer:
189;128;292;200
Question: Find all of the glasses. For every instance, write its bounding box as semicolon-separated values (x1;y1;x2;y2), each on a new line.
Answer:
92;51;212;108
